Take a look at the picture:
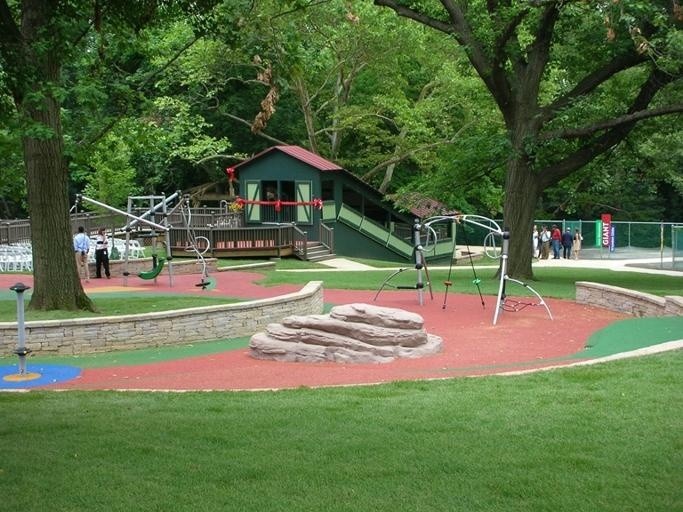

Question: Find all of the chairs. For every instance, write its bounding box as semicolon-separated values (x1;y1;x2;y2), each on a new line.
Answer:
0;237;147;272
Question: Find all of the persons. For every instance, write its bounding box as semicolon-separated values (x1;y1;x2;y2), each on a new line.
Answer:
532;224;539;259
539;227;551;260
572;228;582;260
92;227;110;278
550;224;562;259
72;225;91;280
536;224;545;258
560;227;573;259
